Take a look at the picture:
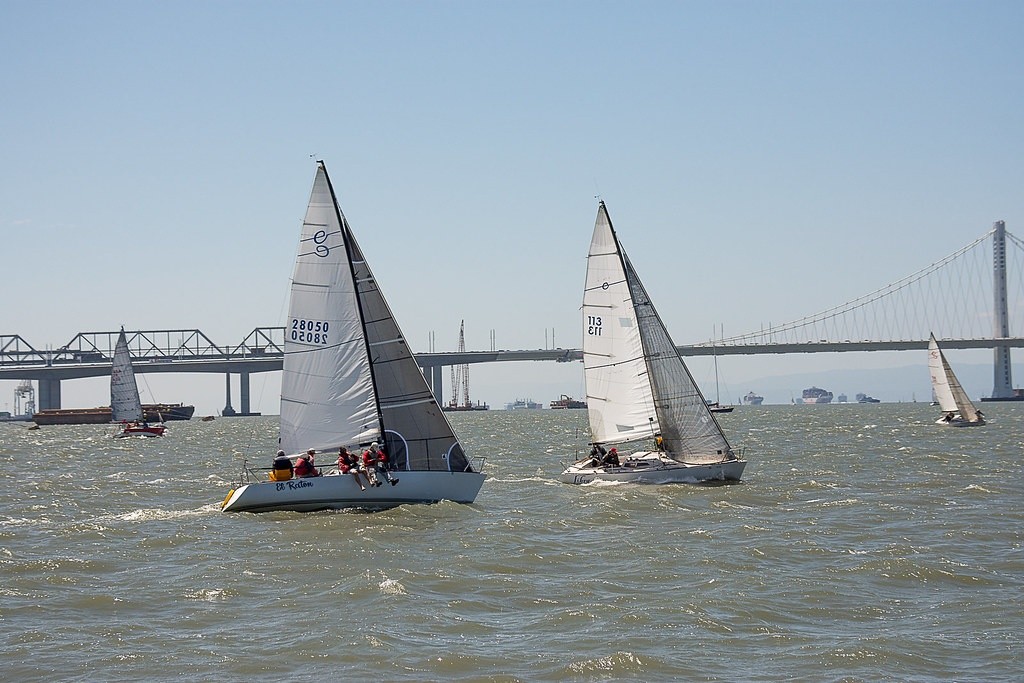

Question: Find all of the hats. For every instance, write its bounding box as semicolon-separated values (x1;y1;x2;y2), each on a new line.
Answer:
307;448;315;454
371;442;379;451
277;450;287;456
339;447;347;455
611;448;617;452
300;454;308;459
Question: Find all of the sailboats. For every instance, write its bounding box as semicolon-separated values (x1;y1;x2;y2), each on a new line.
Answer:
912;392;916;402
556;198;748;485
929;387;939;407
702;354;735;413
791;397;796;406
928;332;986;427
220;157;489;513
110;326;169;440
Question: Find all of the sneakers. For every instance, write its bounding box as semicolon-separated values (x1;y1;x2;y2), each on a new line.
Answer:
390;478;399;487
371;479;378;487
361;485;366;491
376;482;382;487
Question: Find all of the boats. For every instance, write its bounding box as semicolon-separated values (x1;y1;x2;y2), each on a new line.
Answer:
838;393;848;404
743;391;764;406
32;403;194;426
504;397;543;410
801;386;834;404
856;393;881;404
201;416;215;422
550;394;587;410
29;424;41;430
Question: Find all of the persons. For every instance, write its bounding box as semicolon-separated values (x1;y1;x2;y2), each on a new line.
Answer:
942;411;954;422
601;448;620;472
295;448;319;477
123;420;149;433
977;409;985;419
362;442;399;487
589;444;607;468
338;446;375;491
272;450;294;478
